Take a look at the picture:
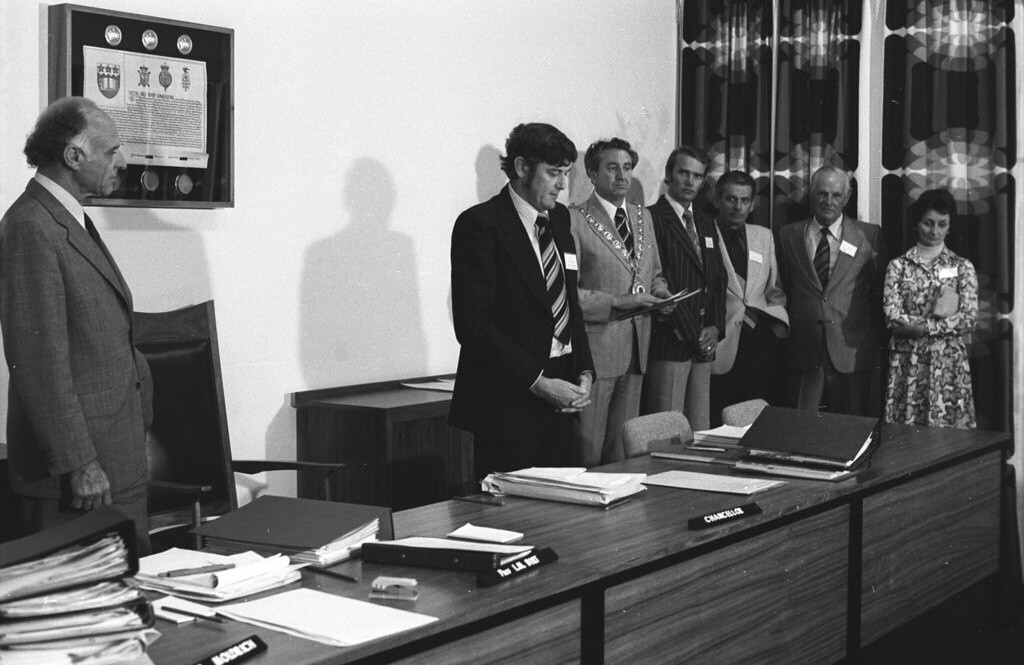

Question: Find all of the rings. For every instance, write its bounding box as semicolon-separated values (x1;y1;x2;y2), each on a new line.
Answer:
708;346;711;350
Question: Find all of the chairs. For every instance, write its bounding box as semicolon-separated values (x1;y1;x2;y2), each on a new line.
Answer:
133;301;344;547
624;399;764;456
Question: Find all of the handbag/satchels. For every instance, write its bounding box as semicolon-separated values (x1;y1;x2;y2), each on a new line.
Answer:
932;285;960;319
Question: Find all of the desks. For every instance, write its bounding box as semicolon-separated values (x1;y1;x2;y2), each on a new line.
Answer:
148;424;1021;665
291;374;462;512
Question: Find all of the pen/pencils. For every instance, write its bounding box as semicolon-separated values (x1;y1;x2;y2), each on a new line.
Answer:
161;606;228;623
605;498;630;511
307;565;360;583
453;495;505;505
158;564;234;577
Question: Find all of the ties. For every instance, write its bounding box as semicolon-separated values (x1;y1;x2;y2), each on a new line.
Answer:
682;210;703;267
813;228;830;288
534;213;573;344
733;232;759;329
615;208;635;259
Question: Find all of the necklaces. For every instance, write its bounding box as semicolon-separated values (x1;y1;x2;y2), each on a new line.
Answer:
568;201;646;294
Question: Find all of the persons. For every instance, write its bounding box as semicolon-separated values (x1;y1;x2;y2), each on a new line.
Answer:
0;96;155;557
647;145;729;431
710;170;791;428
569;135;678;467
451;122;597;495
779;164;890;414
883;190;976;429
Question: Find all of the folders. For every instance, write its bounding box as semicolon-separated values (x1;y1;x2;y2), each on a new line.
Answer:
739;406;881;472
190;496;395;569
